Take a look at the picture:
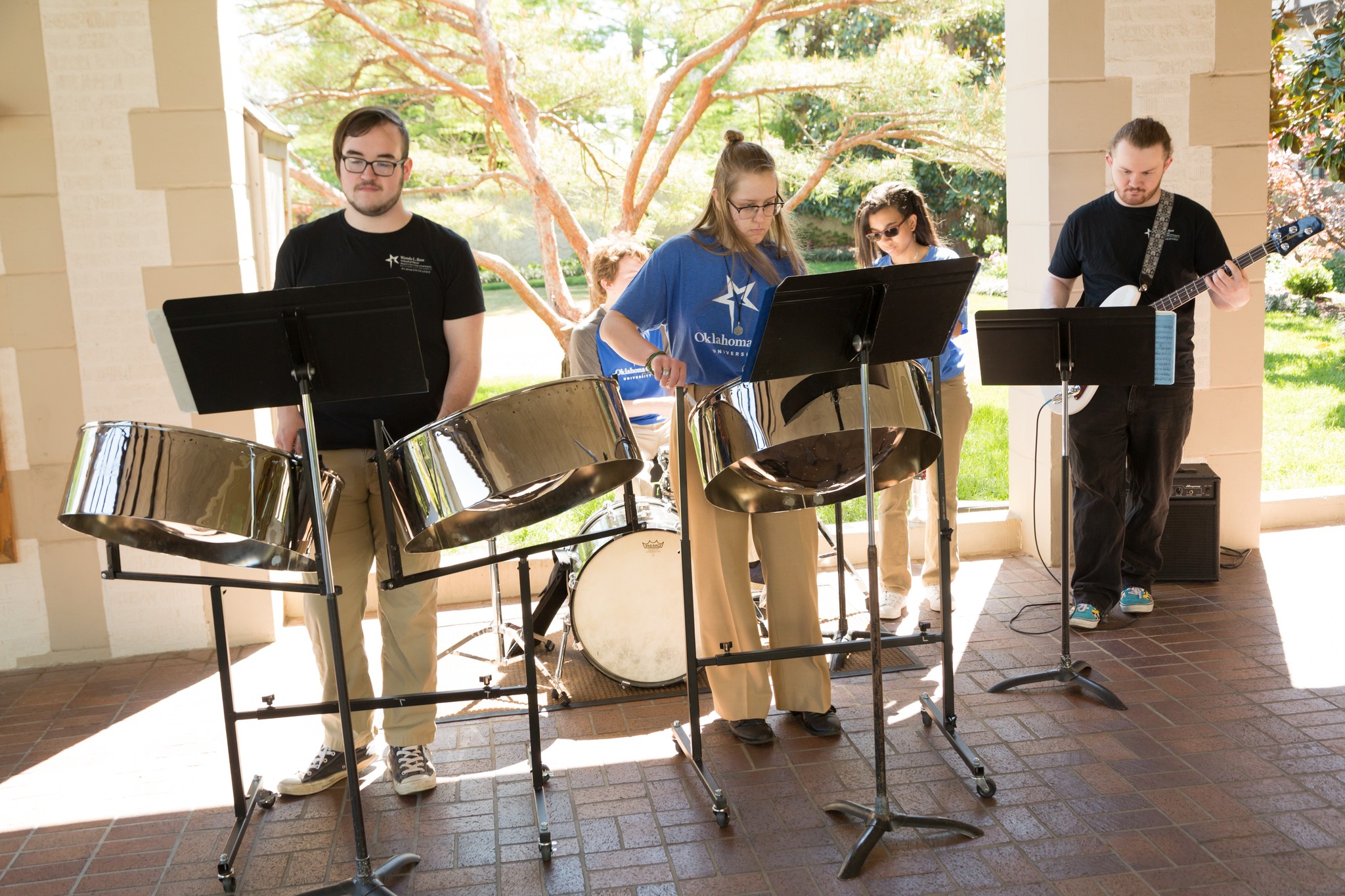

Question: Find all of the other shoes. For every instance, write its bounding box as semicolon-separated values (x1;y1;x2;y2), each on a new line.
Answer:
748;560;767;590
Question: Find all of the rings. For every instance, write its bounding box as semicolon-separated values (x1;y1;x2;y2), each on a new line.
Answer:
663;368;670;376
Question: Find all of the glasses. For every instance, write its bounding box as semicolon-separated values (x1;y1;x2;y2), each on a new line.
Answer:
340;153;406;177
865;215;909;242
726;192;784;220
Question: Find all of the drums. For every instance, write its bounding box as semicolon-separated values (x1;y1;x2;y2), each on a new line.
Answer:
378;376;646;556
56;418;347;573
566;495;709;691
685;356;946;516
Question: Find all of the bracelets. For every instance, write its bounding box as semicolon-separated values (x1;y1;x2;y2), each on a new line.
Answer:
644;351;668;376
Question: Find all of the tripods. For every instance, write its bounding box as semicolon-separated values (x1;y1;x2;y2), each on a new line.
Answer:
976;307;1160;710
751;254;986;881
819;501;900;673
161;275;431;896
435;536;575;706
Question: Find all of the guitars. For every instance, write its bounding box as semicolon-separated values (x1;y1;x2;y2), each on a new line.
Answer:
1048;214;1327;418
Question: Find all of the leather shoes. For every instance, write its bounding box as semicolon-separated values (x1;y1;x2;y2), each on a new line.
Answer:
789;703;842;737
728;718;774;745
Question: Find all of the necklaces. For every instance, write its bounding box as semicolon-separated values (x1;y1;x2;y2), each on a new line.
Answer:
911;242;919;263
719;233;757;336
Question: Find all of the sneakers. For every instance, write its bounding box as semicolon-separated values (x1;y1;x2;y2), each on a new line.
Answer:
924;583;956;612
387;743;437;795
879;590;909;619
276;743;380;796
1068;603;1101;629
1119;586;1154;613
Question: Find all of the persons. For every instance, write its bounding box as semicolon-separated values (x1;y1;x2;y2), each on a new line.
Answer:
570;232;770;590
853;182;973;620
600;131;841;745
270;107;484;794
1047;116;1251;629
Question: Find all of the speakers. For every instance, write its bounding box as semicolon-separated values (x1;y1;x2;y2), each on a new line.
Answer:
1124;463;1221;582
